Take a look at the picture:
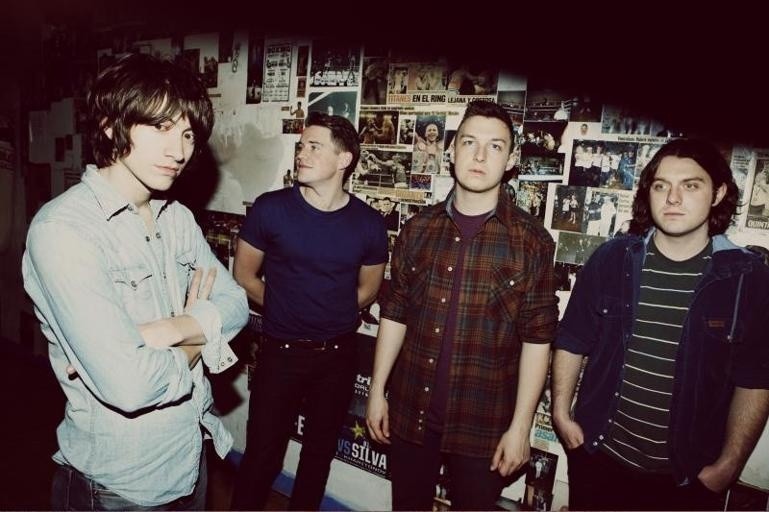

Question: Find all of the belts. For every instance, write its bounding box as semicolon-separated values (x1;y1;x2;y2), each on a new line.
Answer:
278;336;351;350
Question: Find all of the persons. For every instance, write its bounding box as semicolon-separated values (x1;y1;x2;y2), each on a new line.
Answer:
229;115;390;512
306;42;448;214
19;46;252;511
364;89;560;512
548;137;767;512
448;61;479;96
510;50;665;266
